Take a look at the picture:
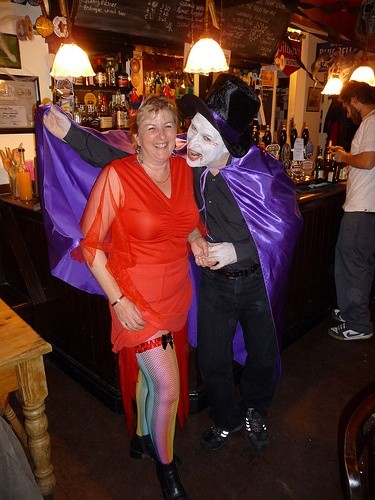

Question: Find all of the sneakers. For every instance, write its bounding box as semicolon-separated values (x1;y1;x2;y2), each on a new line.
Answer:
200;425;243;452
243;408;270;450
332;308;346;322
329;323;373;341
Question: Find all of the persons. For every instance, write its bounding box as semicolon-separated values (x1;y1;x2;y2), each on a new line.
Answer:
325;83;375;339
38;74;302;444
80;96;216;500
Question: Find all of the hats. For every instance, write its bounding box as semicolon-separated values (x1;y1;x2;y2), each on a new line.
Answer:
174;73;261;158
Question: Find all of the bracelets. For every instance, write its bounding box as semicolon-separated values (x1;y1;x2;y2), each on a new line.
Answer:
111;294;124;307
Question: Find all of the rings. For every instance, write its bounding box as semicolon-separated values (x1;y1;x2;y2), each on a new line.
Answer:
125;321;130;325
332;157;335;161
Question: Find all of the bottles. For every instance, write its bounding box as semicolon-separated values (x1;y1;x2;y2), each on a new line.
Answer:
143;68;196;134
17;147;33;201
231;64;271;152
279;122;349;184
70;52;142;151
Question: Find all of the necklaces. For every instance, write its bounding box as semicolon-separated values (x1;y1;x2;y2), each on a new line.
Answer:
140;165;171;183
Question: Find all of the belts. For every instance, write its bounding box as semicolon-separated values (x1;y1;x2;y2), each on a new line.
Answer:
218;263;258;278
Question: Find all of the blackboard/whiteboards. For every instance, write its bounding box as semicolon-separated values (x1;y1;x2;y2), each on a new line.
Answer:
70;0;294;64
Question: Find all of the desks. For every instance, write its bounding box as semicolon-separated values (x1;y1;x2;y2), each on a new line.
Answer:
0;296;56;498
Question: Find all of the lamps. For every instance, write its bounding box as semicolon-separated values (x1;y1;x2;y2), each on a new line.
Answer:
182;0;230;75
320;18;375;95
49;0;96;78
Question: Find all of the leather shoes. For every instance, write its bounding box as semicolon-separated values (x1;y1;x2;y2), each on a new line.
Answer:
156;461;188;500
129;432;182;467
